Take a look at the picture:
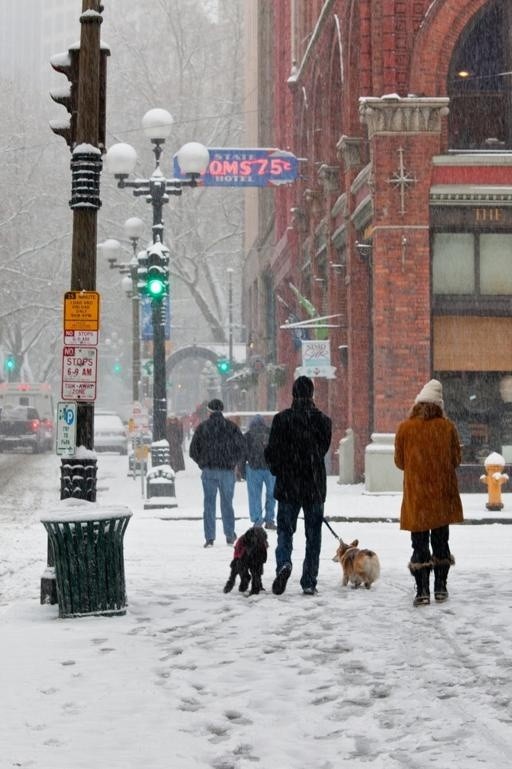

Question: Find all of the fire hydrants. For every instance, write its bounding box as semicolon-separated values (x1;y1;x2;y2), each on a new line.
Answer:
477;451;509;514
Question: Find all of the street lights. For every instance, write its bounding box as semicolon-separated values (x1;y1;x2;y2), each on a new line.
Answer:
123;276;150;396
103;217;152;402
106;107;211;510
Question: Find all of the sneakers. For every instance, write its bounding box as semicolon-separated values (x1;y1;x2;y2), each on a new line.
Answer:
204;540;213;547
271;564;291;594
266;524;277;529
227;532;236;544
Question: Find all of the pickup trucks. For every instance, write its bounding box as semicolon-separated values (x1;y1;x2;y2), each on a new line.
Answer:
1;405;48;452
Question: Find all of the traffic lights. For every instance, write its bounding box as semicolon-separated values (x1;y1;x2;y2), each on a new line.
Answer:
215;359;231;375
5;352;14;372
149;251;164;296
138;250;150;294
48;41;111;150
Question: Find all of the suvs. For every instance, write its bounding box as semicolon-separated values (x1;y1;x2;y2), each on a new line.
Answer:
94;411;128;457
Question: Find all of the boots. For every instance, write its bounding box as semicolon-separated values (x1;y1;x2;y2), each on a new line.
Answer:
408;558;434;606
432;553;455;603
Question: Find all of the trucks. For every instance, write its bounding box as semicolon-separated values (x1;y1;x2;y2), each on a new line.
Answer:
0;383;56;452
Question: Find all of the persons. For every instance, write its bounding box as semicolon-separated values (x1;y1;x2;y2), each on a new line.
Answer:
264;375;333;595
393;379;465;606
128;398;278;547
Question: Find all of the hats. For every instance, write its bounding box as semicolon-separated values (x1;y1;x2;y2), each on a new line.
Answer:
249;414;264;427
293;376;314;399
207;399;224;413
415;379;444;411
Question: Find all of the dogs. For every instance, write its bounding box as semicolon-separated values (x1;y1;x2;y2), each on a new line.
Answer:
224;527;269;595
332;538;381;589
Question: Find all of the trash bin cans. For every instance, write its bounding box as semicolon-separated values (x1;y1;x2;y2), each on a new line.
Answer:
40;502;133;618
